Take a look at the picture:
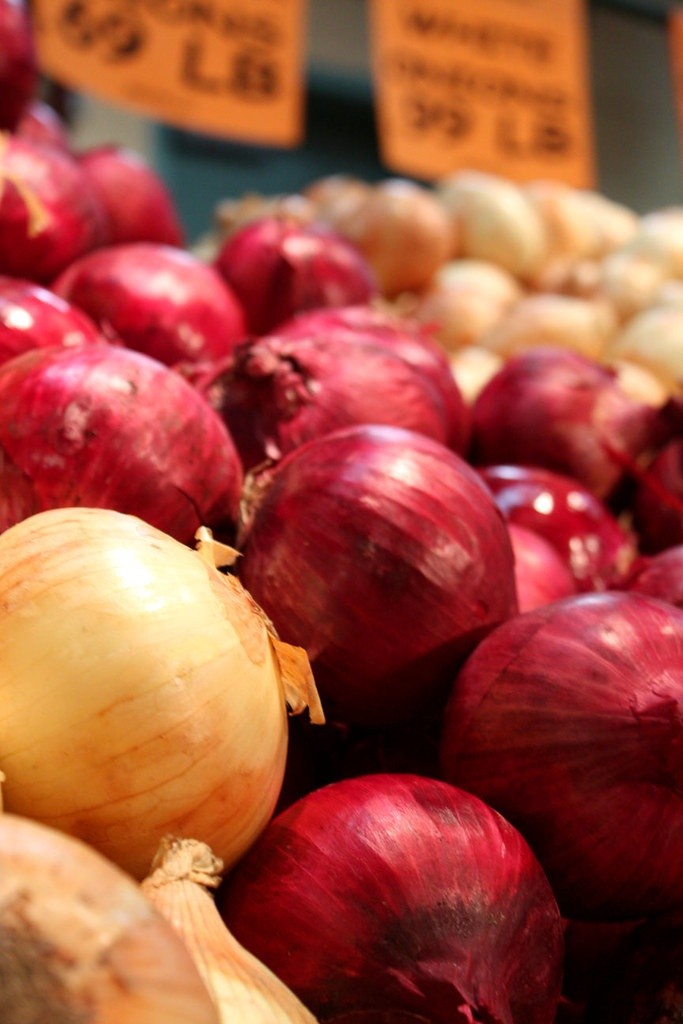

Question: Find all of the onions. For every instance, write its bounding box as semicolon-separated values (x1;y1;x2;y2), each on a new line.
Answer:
0;0;683;1024
